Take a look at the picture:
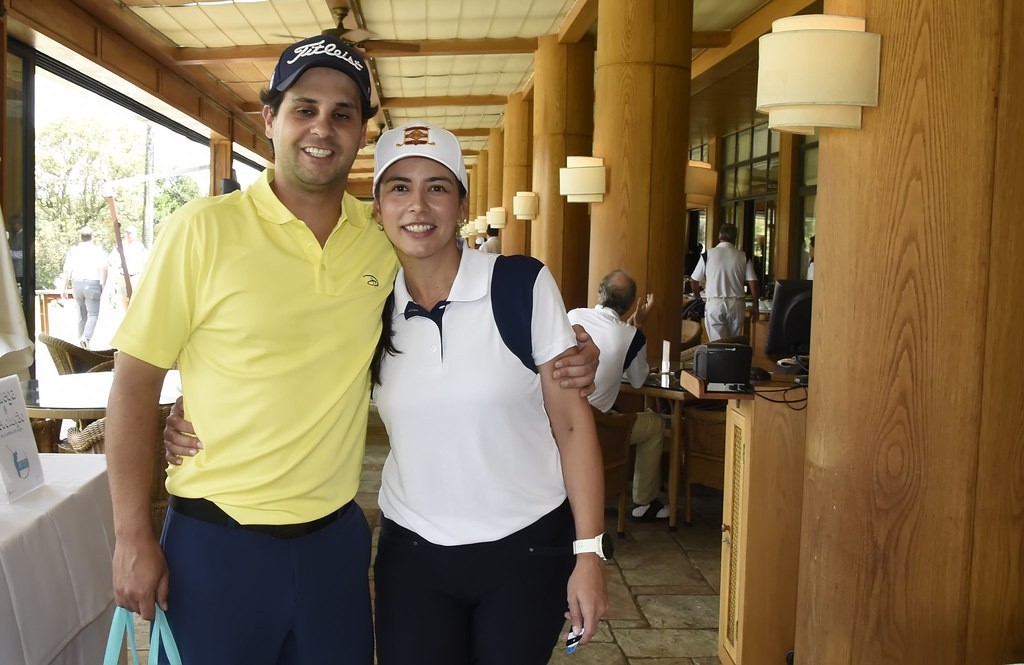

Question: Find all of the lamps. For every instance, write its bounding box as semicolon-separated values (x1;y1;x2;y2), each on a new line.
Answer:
559;156;606;204
756;13;882;135
460;216;486;239
684;160;717;209
486;207;505;228
513;191;539;220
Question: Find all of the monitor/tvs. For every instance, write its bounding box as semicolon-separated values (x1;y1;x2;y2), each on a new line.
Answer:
765;279;813;360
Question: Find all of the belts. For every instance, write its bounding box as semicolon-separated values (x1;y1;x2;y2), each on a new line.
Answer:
170;492;351;539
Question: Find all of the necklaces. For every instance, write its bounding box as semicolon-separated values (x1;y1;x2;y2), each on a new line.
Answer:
406;275;454;308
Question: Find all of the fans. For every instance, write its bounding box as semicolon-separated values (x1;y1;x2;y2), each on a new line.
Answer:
366;122;385;147
272;6;420;53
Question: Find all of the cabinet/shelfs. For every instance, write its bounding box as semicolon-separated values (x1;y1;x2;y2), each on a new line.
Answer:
679;368;808;665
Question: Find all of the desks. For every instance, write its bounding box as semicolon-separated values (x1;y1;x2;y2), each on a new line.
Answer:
0;453;128;665
621;362;696;533
35;289;74;335
21;370;181;419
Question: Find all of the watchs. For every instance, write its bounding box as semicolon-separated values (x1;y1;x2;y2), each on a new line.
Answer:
572;532;614;562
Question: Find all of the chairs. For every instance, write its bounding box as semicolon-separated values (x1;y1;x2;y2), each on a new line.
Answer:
30;333;179;545
591;319;727;540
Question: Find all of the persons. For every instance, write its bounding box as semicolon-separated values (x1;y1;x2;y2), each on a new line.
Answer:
691;223;760;344
61;226;109;351
807;236;814;281
110;225;146;311
105;33;603;665
163;123;611;665
567;269;672;520
478;225;502;255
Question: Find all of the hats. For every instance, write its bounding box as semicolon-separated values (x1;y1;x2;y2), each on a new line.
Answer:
269;35;372;106
372;121;468;199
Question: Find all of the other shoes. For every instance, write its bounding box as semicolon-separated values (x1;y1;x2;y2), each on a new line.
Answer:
80;337;89;350
633;500;671;521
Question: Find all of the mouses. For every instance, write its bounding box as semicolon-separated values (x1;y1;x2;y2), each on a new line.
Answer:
750;367;771;380
